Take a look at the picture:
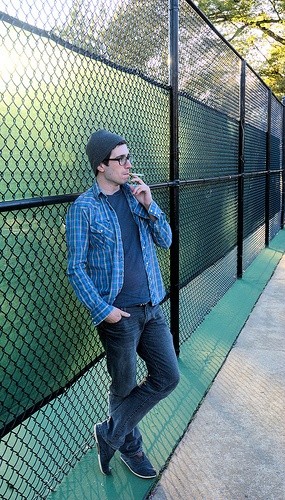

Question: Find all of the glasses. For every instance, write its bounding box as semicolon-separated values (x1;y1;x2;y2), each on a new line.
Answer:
105;155;133;166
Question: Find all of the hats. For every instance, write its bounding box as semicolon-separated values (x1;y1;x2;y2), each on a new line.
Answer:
86;129;125;174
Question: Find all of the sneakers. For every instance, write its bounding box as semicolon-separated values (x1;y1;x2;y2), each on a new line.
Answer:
120;450;156;478
94;423;116;475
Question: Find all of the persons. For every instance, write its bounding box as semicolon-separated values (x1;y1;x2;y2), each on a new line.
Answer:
63;129;181;480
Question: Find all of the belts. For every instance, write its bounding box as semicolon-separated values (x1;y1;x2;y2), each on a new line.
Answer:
126;302;148;308
